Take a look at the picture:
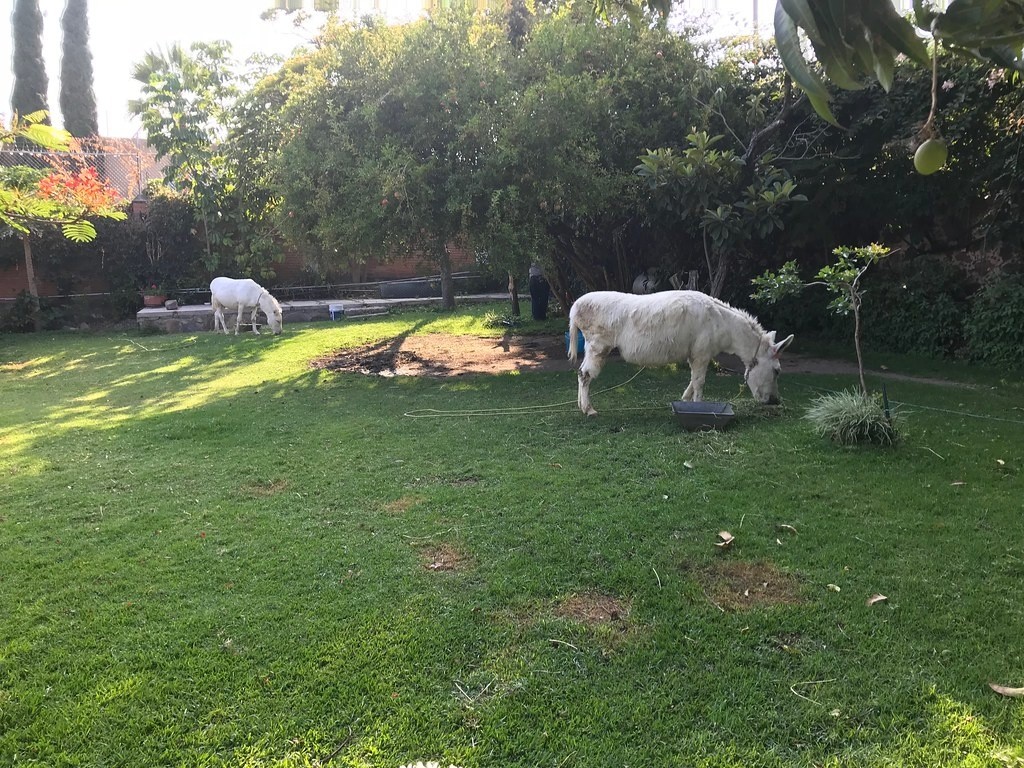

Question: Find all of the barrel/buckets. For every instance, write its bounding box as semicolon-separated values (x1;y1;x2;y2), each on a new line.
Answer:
328;304;343;320
564;331;584;353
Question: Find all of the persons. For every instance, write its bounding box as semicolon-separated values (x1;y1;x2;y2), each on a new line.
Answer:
529;262;549;320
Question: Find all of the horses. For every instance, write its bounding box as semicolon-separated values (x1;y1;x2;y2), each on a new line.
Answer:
566;290;794;417
209;276;283;337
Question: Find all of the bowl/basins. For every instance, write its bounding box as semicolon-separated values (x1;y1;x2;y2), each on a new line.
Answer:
671;401;736;431
234;323;261;333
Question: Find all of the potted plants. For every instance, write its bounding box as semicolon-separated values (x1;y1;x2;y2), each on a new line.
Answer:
143;284;165;307
